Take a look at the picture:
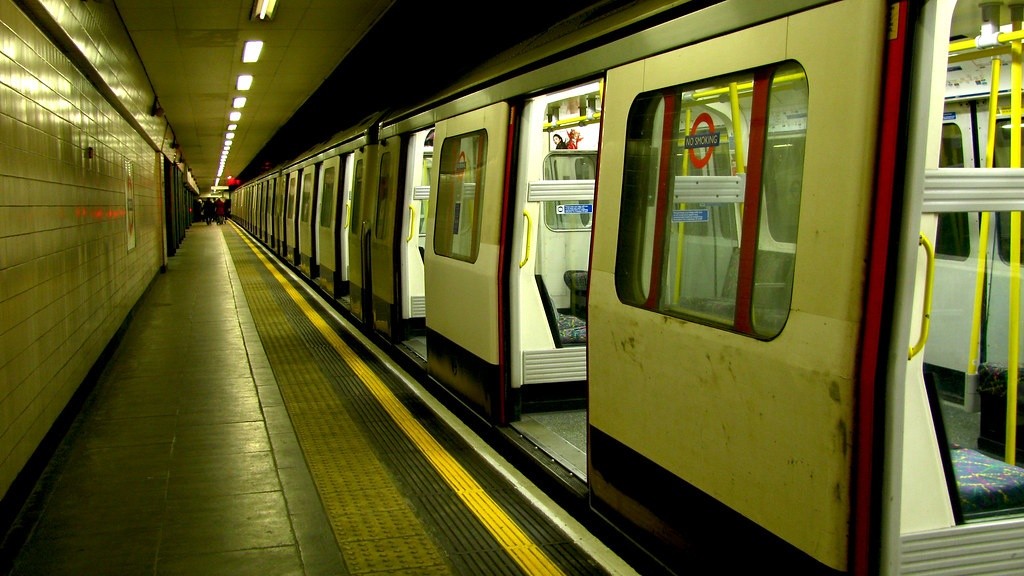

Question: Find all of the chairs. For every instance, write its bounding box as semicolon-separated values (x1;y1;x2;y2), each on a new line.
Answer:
534;273;586;347
921;363;1024;522
693;246;790;325
761;256;796;328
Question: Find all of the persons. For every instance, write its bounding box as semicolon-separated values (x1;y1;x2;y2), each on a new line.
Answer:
223;199;230;220
214;198;224;225
203;197;214;226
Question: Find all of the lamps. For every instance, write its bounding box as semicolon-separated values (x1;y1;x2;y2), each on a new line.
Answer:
250;0;280;24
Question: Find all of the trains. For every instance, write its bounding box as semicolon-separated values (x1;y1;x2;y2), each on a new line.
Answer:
229;0;1024;576
